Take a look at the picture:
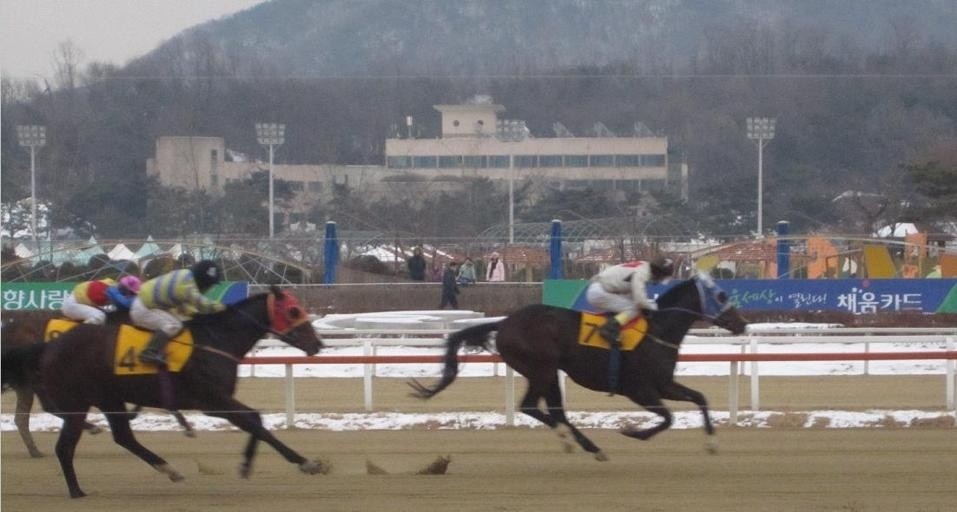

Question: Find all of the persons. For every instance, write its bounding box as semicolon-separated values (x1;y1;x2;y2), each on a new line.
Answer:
585;257;674;345
438;261;461;310
129;259;228;366
61;274;143;328
457;258;477;288
406;247;427;282
486;252;505;282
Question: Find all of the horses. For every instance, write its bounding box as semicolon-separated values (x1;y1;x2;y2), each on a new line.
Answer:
0;281;332;502
404;271;751;466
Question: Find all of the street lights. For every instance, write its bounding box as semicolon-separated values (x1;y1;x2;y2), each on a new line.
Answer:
494;119;525;247
744;116;778;242
254;121;286;246
15;123;46;265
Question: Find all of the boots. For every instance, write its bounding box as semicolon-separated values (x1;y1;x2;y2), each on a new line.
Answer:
599;318;622;348
139;328;168;370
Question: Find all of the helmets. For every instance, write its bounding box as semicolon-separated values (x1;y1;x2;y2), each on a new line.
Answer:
194;260;221;283
121;275;141;294
653;258;674;274
